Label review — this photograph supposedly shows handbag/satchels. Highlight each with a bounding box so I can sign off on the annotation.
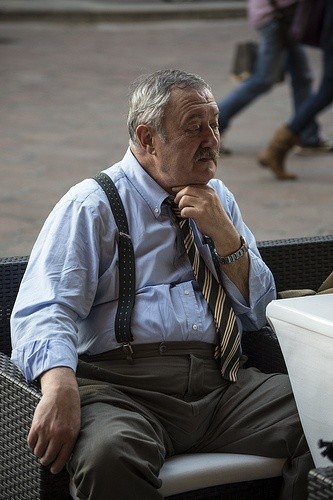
[230,42,286,83]
[290,0,325,48]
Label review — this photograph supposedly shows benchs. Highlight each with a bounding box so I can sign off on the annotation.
[0,231,332,500]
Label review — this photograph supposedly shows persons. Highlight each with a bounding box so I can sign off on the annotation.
[215,0,333,182]
[9,69,316,500]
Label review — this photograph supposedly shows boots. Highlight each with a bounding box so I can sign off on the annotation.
[256,123,297,180]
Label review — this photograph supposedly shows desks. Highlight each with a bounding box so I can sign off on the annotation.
[265,293,333,471]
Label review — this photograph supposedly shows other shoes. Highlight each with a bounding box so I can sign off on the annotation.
[295,140,333,155]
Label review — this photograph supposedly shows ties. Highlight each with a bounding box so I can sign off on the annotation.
[166,196,242,383]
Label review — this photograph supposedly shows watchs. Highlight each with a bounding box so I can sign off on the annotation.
[213,234,249,264]
